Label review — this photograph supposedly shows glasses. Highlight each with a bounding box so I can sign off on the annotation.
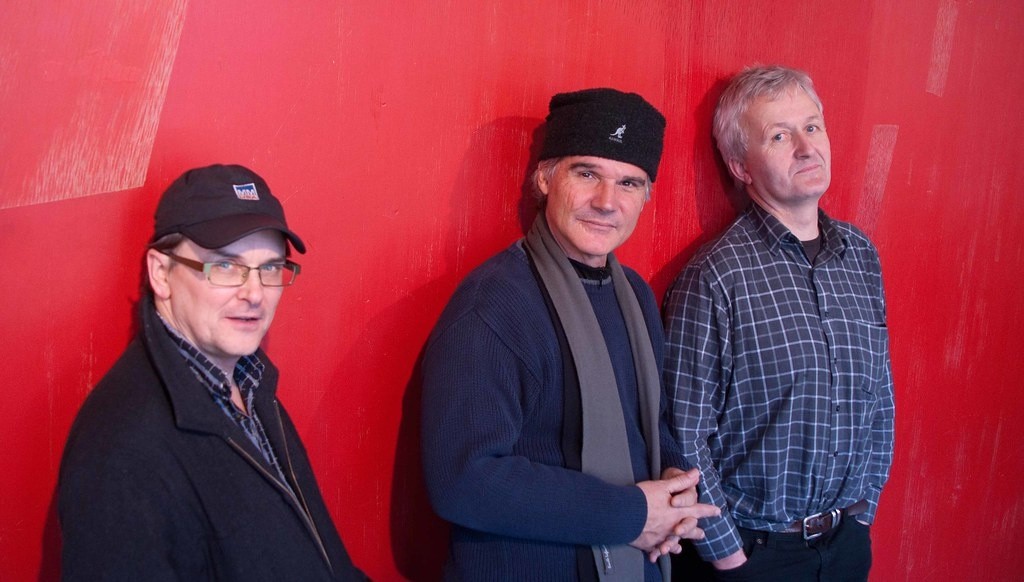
[160,251,301,287]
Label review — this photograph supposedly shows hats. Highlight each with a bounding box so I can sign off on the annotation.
[538,85,666,185]
[155,164,308,255]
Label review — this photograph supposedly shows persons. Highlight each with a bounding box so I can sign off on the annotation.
[56,162,373,582]
[664,66,896,582]
[391,86,722,582]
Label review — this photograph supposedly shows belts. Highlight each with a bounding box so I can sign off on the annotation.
[779,500,870,541]
